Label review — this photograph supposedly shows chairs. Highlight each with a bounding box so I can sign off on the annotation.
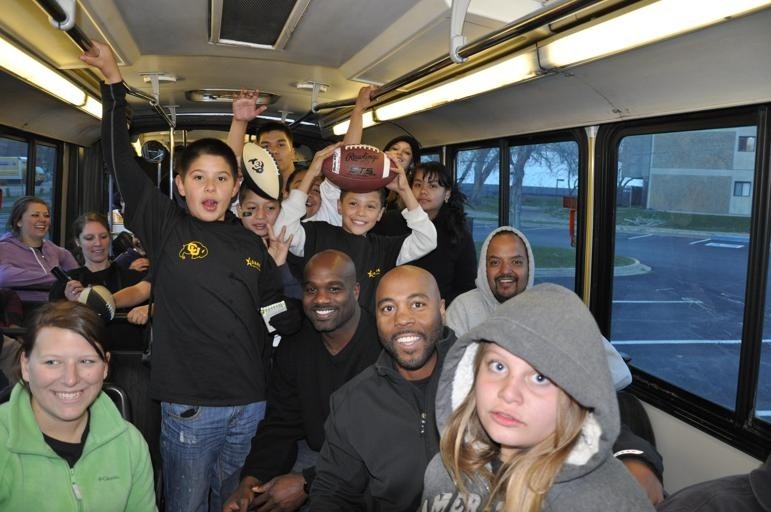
[1,348,162,512]
[110,313,151,351]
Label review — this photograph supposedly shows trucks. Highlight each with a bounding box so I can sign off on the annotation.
[1,157,45,186]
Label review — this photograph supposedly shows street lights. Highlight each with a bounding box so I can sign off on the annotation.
[554,177,565,201]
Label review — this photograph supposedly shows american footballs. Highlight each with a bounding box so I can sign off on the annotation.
[321,145,398,193]
[240,139,282,204]
[79,283,116,326]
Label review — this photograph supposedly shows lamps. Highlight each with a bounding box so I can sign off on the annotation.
[0,36,87,110]
[536,1,771,77]
[80,93,103,121]
[373,45,554,129]
[333,108,383,141]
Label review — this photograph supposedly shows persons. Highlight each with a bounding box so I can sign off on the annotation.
[656,454,771,512]
[598,328,664,507]
[420,282,658,512]
[444,224,535,341]
